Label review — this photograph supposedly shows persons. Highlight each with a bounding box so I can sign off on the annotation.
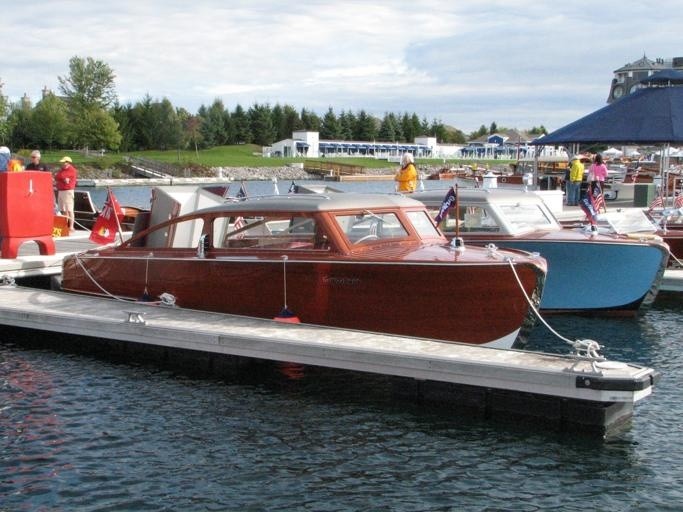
[568,155,587,206]
[589,154,608,194]
[25,149,49,171]
[0,143,11,171]
[55,156,77,232]
[395,152,417,194]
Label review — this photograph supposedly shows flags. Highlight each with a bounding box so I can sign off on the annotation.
[235,216,245,239]
[674,196,683,208]
[649,191,662,210]
[89,191,125,246]
[580,180,604,224]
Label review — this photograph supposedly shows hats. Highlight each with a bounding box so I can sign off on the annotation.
[571,156,578,160]
[59,156,72,163]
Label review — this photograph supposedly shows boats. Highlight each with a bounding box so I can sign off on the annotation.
[58,184,548,354]
[354,188,672,316]
